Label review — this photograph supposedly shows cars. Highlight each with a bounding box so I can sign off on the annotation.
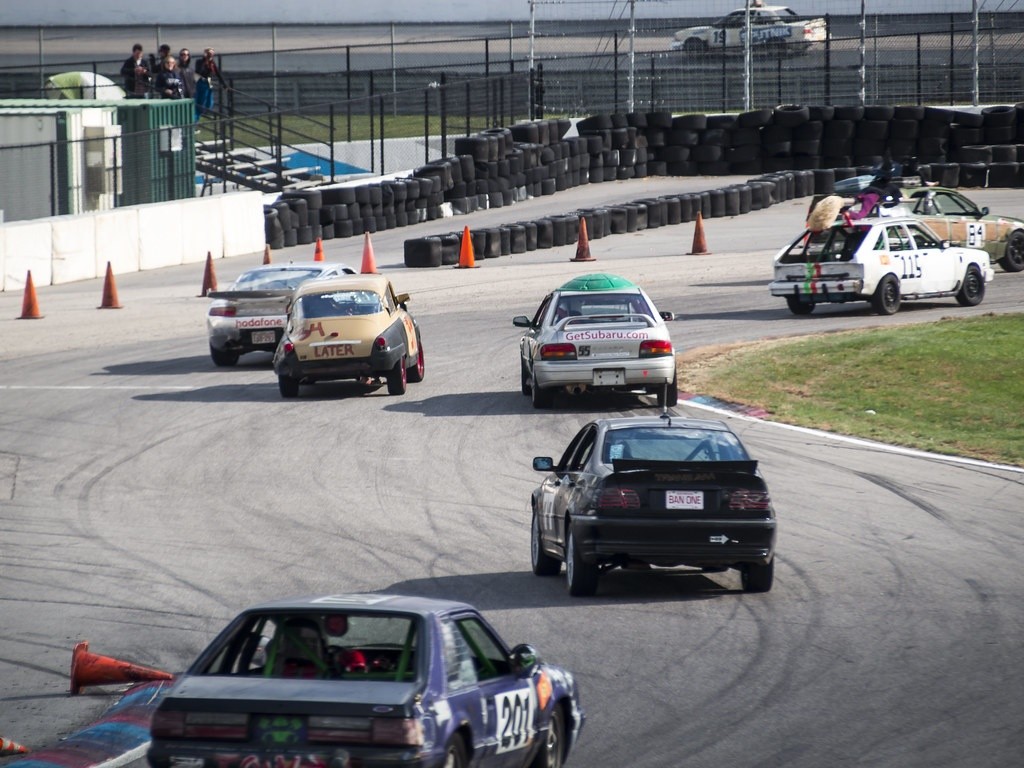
[512,288,678,407]
[201,260,358,367]
[672,0,833,58]
[272,272,424,398]
[145,593,587,766]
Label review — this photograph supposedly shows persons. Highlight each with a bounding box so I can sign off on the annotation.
[121,44,218,122]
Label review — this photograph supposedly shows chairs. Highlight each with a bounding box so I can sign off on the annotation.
[312,299,339,316]
[557,311,583,324]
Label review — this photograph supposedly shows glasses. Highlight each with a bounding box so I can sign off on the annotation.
[180,53,189,56]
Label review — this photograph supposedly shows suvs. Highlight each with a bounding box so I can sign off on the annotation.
[534,415,777,597]
[807,187,1023,272]
[768,218,995,316]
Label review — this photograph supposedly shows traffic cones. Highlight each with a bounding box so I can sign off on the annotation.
[69,641,172,697]
[96,261,124,309]
[314,236,323,261]
[454,226,481,268]
[262,243,272,265]
[15,269,45,320]
[196,251,218,297]
[360,231,377,274]
[685,210,712,256]
[568,217,597,261]
[0,737,31,756]
